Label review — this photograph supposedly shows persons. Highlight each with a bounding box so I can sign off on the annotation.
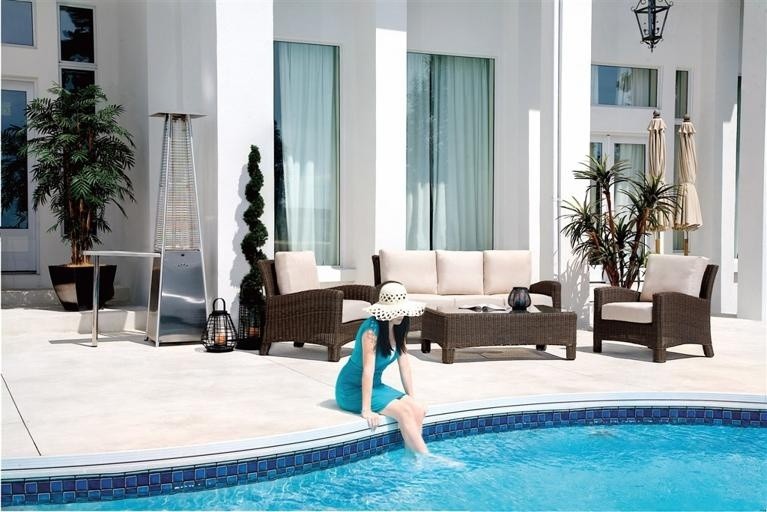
[336,282,466,468]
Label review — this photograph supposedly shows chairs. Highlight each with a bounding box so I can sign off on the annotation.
[591,247,719,367]
[256,249,383,360]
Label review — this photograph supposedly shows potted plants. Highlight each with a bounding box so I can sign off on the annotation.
[4,76,133,310]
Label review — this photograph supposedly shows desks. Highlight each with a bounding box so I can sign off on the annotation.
[81,245,162,347]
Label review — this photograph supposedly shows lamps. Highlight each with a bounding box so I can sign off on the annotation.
[630,1,675,54]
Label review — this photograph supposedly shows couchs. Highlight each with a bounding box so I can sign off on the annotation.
[373,248,562,344]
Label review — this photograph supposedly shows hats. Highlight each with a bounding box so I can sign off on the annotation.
[363,283,427,322]
